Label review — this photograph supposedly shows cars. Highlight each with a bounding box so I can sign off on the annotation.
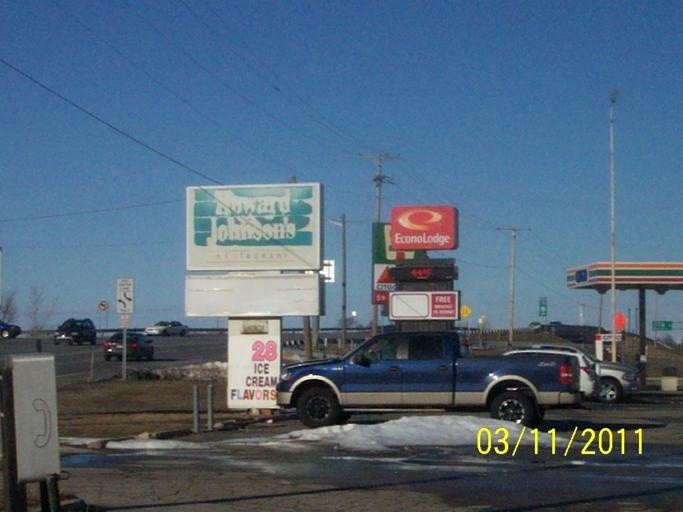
[104,333,154,361]
[145,319,190,337]
[504,342,643,403]
[0,319,21,338]
[55,318,97,346]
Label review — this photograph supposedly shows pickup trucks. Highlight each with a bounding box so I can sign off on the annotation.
[277,330,579,427]
